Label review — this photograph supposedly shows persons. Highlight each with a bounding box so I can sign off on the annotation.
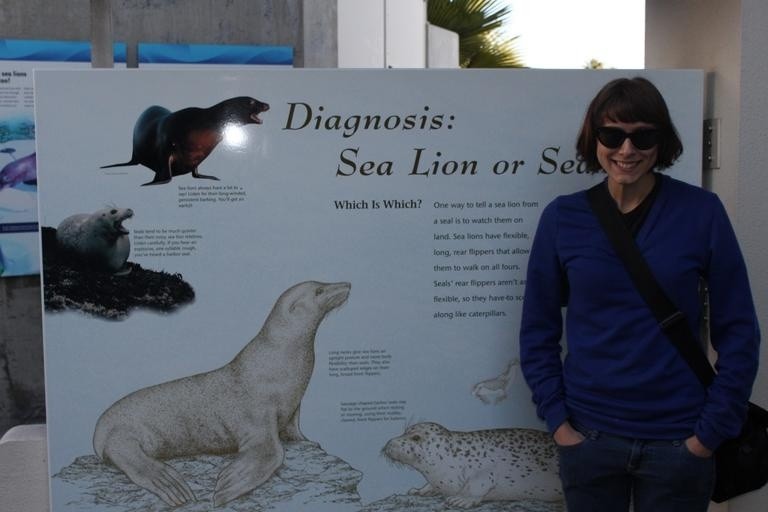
[516,76,765,511]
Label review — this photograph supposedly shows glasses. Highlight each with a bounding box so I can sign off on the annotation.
[595,125,657,150]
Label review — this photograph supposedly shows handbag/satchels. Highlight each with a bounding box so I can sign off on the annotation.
[710,398,768,504]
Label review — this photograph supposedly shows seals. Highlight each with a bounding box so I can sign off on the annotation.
[383,421,566,510]
[54,207,134,286]
